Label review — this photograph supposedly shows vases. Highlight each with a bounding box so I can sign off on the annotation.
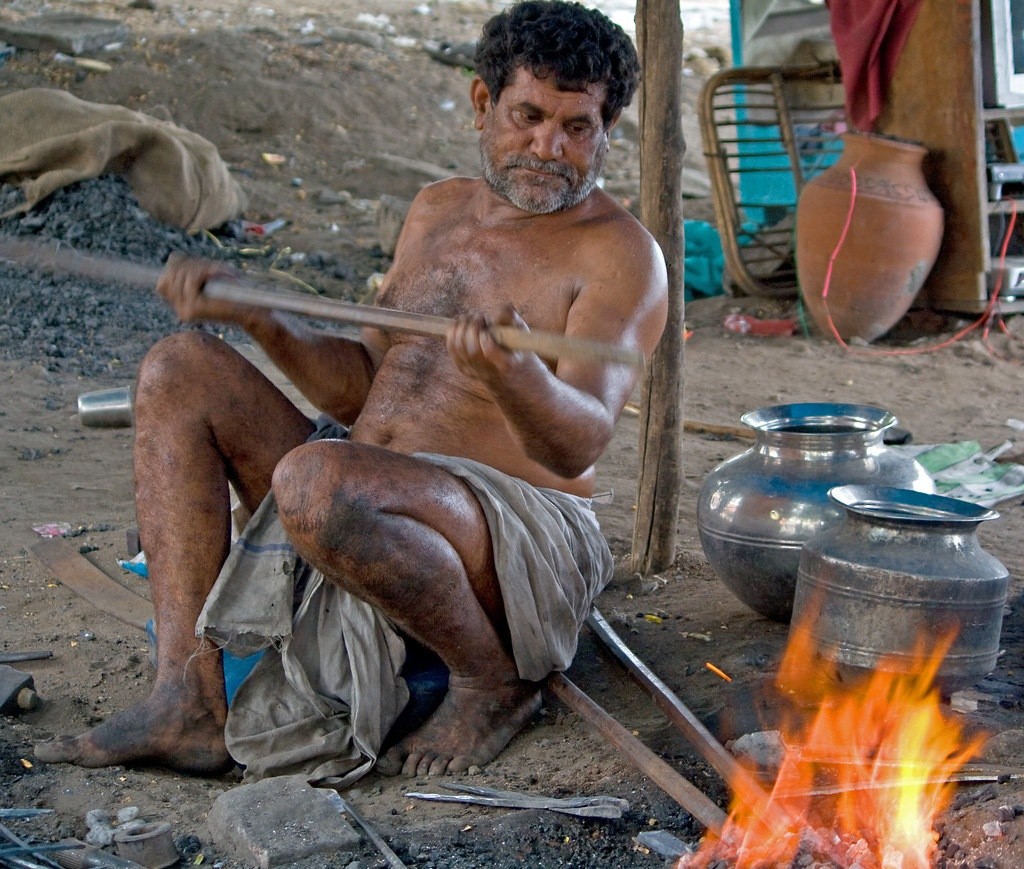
[697,403,938,625]
[791,484,1010,698]
[794,132,945,343]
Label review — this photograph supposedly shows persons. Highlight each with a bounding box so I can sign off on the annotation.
[32,0,669,778]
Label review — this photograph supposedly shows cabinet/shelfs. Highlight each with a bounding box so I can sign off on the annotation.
[825,0,1024,313]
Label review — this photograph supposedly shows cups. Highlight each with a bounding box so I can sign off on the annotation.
[78,386,133,427]
